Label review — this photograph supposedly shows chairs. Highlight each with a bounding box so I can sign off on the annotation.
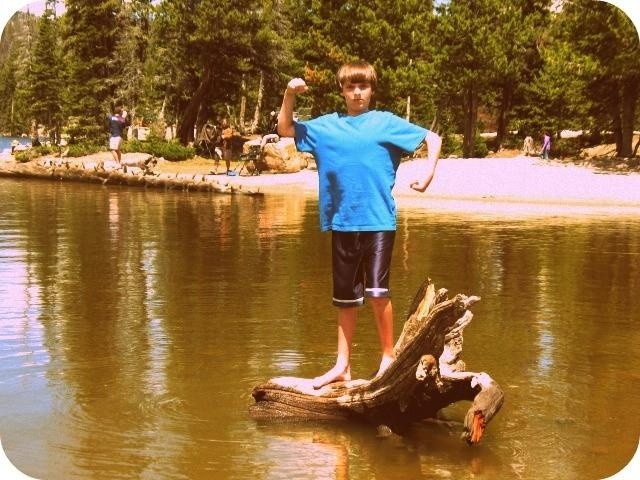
[198,123,221,159]
[237,142,262,177]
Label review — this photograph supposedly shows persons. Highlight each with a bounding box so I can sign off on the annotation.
[259,111,279,152]
[210,117,233,175]
[541,131,551,161]
[109,107,127,170]
[277,60,443,390]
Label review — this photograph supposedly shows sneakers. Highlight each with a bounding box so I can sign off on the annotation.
[210,169,218,176]
[112,162,125,170]
